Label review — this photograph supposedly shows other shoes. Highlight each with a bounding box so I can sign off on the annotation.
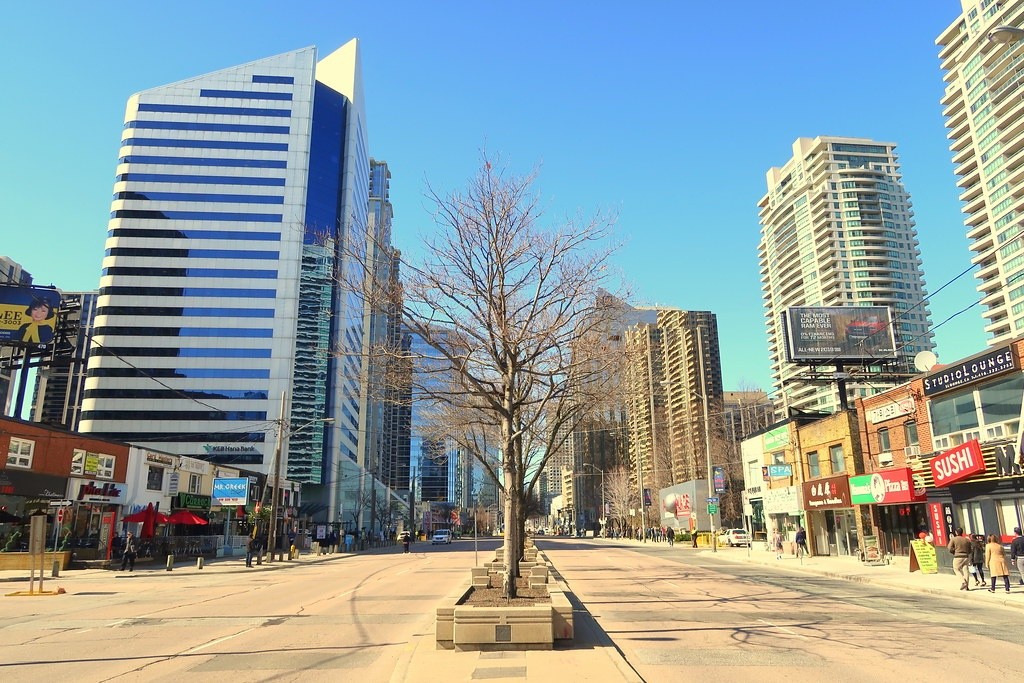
[1006,590,1010,594]
[960,582,967,590]
[975,580,980,586]
[964,586,969,591]
[981,581,986,586]
[988,589,995,593]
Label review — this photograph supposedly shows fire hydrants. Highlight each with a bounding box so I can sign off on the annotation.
[290,544,296,559]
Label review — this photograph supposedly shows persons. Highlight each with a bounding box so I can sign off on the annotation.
[647,527,674,547]
[691,527,697,548]
[348,527,372,544]
[111,532,120,559]
[969,534,986,586]
[874,477,884,501]
[985,534,1010,594]
[403,533,412,554]
[948,527,971,591]
[775,529,782,560]
[796,527,812,558]
[246,533,254,567]
[1011,527,1024,585]
[289,527,296,551]
[607,527,642,542]
[383,528,396,541]
[120,531,137,571]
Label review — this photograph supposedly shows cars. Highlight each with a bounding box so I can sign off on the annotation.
[431,528,453,545]
[722,528,753,548]
[397,530,411,544]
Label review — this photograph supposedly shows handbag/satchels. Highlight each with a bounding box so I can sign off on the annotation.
[968,564,976,573]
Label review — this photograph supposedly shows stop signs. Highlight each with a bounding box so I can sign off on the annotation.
[57,509,64,522]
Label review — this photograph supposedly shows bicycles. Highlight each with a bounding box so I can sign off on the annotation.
[797,542,805,565]
[775,543,782,560]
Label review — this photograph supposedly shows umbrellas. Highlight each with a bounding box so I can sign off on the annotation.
[120,510,168,523]
[140,502,154,539]
[169,510,208,525]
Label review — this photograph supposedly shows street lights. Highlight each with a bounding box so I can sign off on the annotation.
[265,417,336,559]
[410,467,439,544]
[582,462,606,540]
[609,431,647,544]
[659,380,717,553]
